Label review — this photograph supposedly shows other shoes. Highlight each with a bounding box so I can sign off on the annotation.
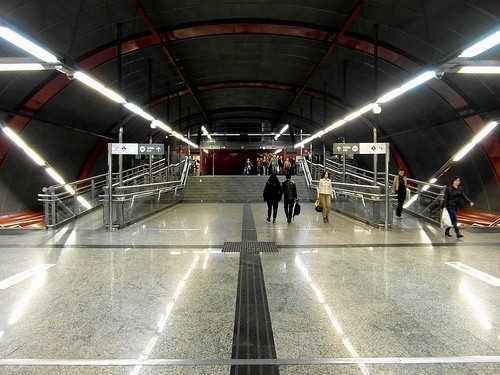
[266,217,271,222]
[456,231,463,238]
[395,215,403,219]
[273,219,276,223]
[287,217,292,223]
[257,174,261,176]
[445,230,452,236]
[323,216,329,223]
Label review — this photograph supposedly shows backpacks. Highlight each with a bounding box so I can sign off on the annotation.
[293,201,301,222]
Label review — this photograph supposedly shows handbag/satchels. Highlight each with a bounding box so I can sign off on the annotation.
[441,208,452,228]
[315,205,322,212]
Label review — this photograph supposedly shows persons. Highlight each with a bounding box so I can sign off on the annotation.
[244,154,297,175]
[393,168,407,218]
[263,173,298,224]
[441,176,474,239]
[319,171,333,224]
